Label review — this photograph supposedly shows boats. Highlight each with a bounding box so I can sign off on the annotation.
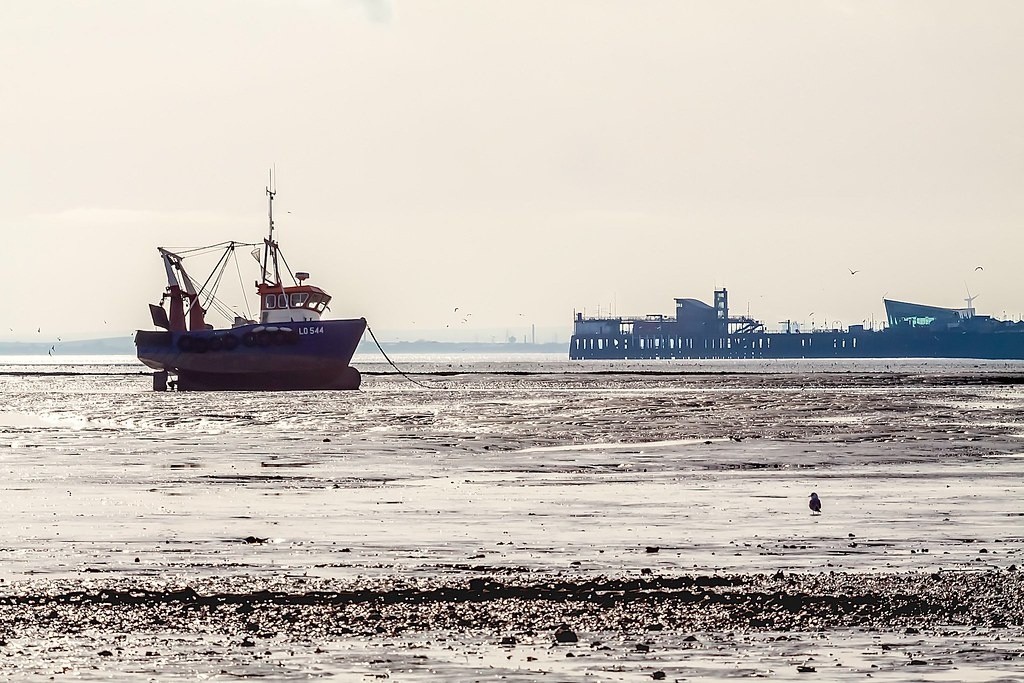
[131,164,372,391]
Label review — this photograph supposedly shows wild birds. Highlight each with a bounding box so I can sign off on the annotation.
[808,493,822,515]
